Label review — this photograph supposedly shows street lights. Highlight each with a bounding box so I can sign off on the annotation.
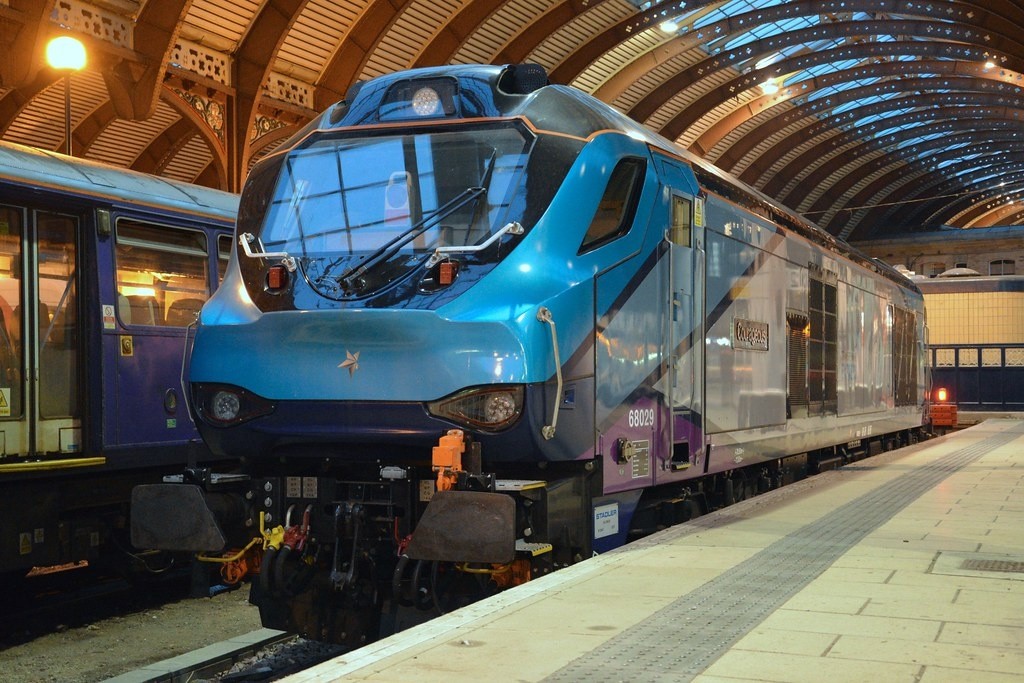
[47,37,88,155]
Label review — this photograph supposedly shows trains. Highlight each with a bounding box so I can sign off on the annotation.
[184,64,932,643]
[0,138,242,609]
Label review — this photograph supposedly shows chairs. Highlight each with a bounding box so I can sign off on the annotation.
[14,290,201,340]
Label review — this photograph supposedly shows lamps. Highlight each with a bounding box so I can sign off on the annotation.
[764,77,778,92]
[662,19,680,32]
